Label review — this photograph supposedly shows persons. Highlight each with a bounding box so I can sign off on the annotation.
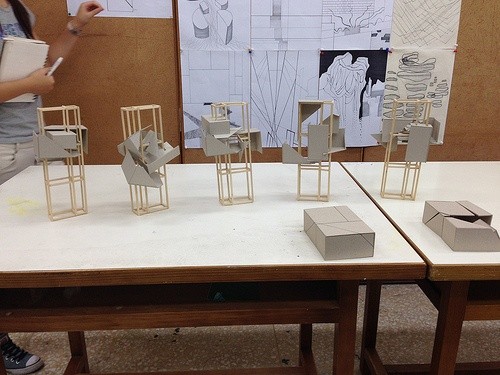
[0,0,105,375]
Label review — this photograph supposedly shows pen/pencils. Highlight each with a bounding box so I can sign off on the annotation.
[47,57,63,76]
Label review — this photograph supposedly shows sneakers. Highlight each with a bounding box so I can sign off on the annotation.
[0,333,43,374]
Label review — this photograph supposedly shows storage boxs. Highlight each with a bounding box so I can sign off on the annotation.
[422,199,500,252]
[303,205,376,260]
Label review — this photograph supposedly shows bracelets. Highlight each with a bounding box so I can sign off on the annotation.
[68,21,81,35]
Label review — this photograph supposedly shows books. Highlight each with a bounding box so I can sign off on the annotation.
[0,34,50,102]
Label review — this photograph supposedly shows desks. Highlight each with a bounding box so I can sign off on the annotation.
[0,160,500,375]
